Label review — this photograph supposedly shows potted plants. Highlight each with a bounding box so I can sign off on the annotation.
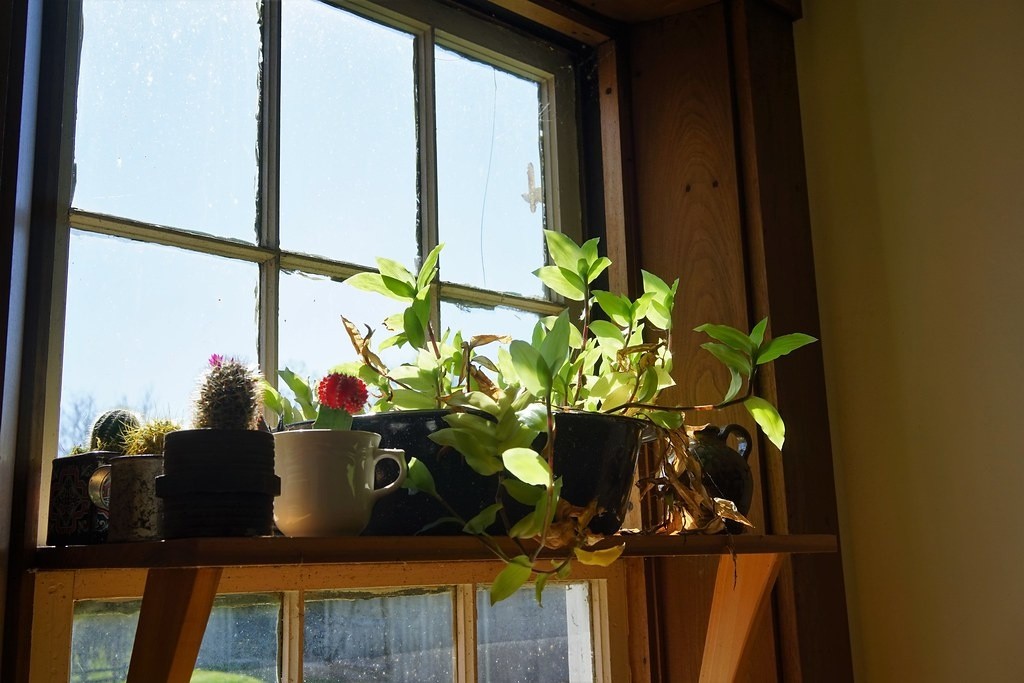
[46,230,820,609]
